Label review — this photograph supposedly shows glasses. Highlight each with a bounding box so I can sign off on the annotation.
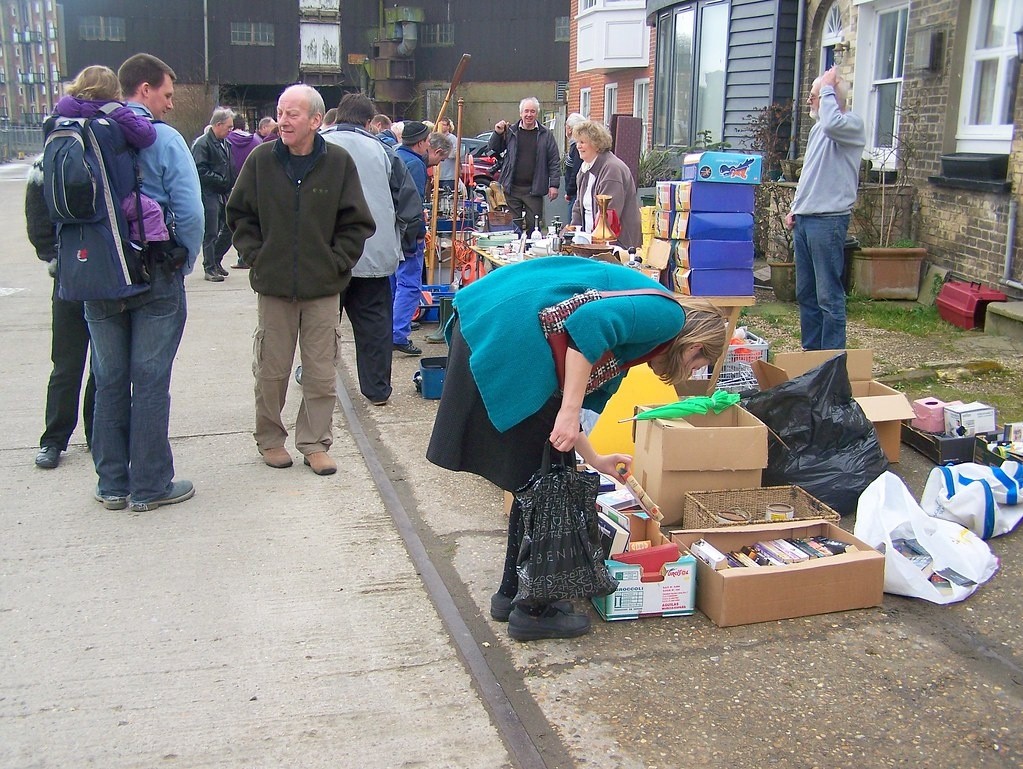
[807,95,820,102]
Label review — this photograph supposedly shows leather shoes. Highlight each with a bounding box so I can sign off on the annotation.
[392,340,422,354]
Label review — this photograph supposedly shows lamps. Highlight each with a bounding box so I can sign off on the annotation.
[831,42,852,65]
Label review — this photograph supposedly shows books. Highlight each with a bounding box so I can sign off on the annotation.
[691,536,852,569]
[584,469,678,582]
[619,468,664,521]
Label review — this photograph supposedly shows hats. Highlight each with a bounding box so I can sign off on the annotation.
[402,122,430,144]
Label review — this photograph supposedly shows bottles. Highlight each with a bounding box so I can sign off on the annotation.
[562,233,574,256]
[530,215,562,252]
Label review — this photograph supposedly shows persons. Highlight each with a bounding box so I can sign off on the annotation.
[489,98,560,231]
[786,66,866,351]
[565,113,588,224]
[392,118,458,354]
[192,109,236,282]
[318,93,423,404]
[227,114,260,268]
[85,53,205,512]
[427,256,727,638]
[225,85,377,474]
[24,65,121,468]
[572,122,641,249]
[254,117,280,143]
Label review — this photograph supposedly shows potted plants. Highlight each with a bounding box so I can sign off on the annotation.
[738,97,804,301]
[847,85,930,302]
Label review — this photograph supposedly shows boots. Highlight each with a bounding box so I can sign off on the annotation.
[424,297,453,343]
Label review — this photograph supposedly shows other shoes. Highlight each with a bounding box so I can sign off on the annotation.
[295,366,302,386]
[216,263,229,276]
[230,262,243,268]
[205,272,224,282]
[508,606,592,640]
[491,592,573,622]
[130,239,151,284]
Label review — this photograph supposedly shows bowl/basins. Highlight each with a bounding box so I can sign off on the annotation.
[571,244,614,257]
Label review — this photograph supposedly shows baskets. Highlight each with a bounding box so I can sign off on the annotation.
[716,332,769,378]
[681,485,841,528]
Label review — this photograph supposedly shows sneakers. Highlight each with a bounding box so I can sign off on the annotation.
[258,444,293,468]
[130,480,195,512]
[94,484,127,509]
[36,446,61,468]
[304,452,337,475]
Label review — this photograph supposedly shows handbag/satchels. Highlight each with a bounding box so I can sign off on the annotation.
[511,439,619,605]
[854,472,996,604]
[538,289,687,398]
[919,460,1023,541]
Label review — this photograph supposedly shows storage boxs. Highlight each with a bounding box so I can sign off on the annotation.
[585,349,1021,630]
[437,219,471,231]
[934,278,1006,329]
[634,150,762,297]
[418,283,457,323]
